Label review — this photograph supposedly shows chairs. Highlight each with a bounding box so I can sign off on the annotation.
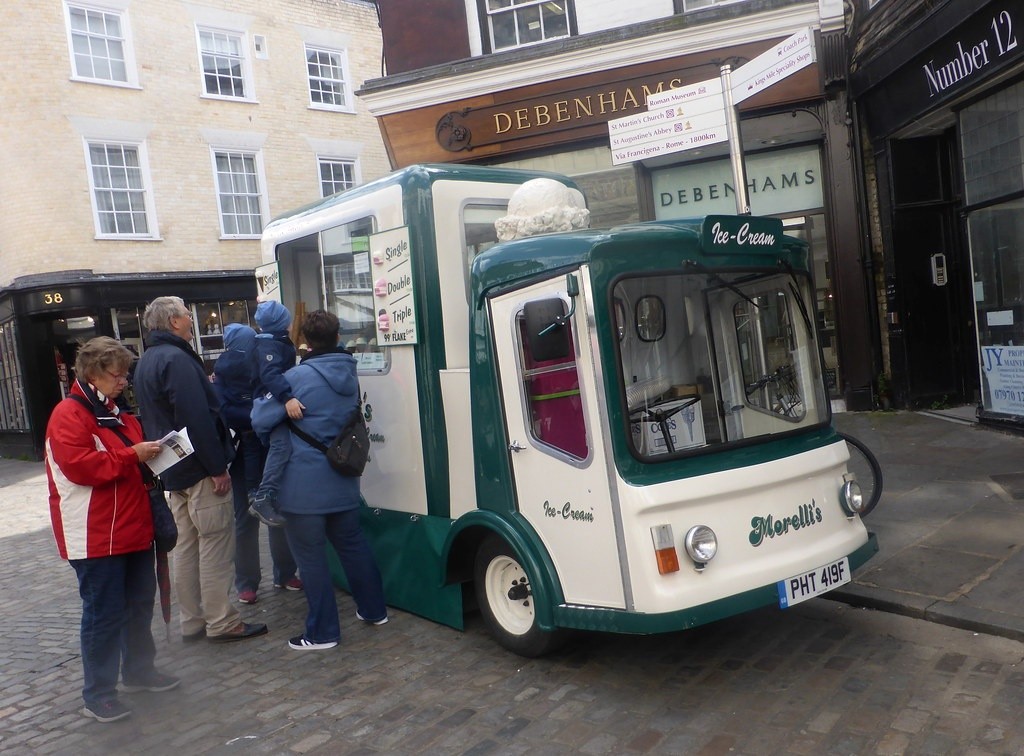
[529,317,587,459]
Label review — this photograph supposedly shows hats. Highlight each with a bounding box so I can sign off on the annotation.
[254,300,292,332]
[223,323,258,351]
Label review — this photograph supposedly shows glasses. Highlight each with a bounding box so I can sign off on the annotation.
[102,366,129,383]
[184,311,193,319]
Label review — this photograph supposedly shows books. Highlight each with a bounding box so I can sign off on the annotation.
[145,427,194,475]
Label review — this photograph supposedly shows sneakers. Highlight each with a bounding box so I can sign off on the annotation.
[248,489,288,527]
[247,489,280,510]
[121,666,181,692]
[356,608,388,625]
[83,692,132,722]
[274,576,303,591]
[238,585,258,603]
[288,633,340,650]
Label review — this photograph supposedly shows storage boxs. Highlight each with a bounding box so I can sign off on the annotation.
[630,383,720,457]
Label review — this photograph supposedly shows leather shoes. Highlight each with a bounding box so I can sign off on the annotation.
[182,621,206,641]
[207,622,268,643]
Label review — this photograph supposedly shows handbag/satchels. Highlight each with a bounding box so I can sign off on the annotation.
[148,487,178,552]
[325,409,371,477]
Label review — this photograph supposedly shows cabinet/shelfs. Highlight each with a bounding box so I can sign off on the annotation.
[199,334,228,361]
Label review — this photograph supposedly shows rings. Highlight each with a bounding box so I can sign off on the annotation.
[220,485,225,488]
[152,453,155,458]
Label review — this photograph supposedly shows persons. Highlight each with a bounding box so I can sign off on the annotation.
[44,335,183,722]
[209,323,302,603]
[215,301,305,528]
[133,296,269,641]
[250,309,388,650]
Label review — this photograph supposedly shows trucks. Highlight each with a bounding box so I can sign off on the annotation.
[256,161,882,661]
